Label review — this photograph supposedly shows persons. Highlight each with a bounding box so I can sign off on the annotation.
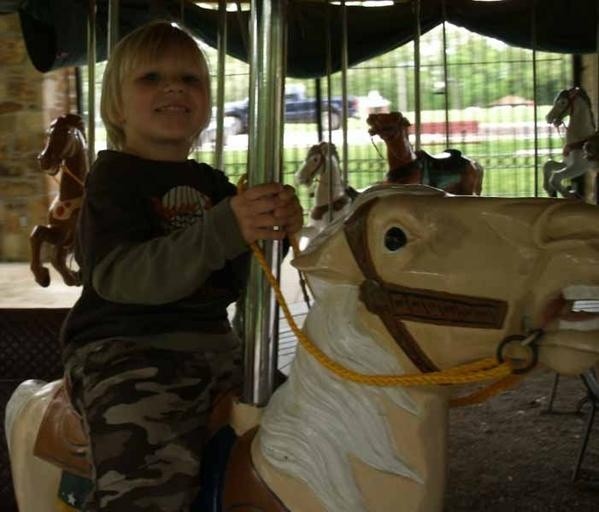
[55,17,307,511]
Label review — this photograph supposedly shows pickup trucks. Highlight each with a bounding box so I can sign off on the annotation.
[223,86,358,135]
